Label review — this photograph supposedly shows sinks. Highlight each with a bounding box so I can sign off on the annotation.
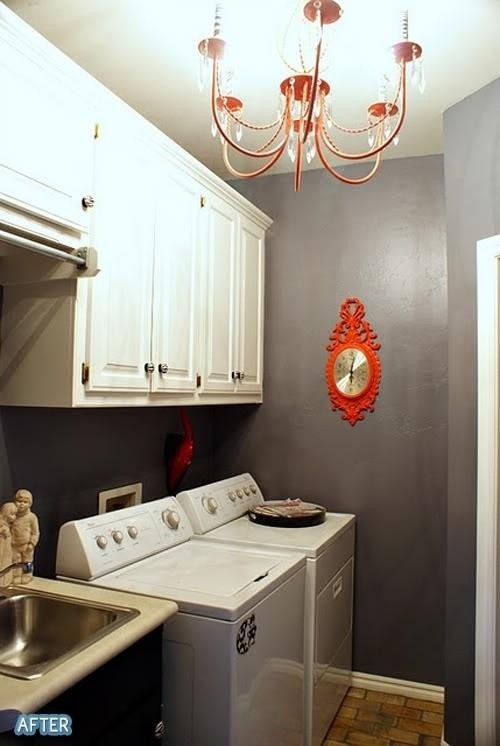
[0,584,142,682]
[0,561,34,578]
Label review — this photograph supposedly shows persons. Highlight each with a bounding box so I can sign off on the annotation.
[9,486,40,585]
[0,501,18,588]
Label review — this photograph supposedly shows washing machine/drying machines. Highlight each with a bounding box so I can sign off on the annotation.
[175,473,358,746]
[55,496,308,746]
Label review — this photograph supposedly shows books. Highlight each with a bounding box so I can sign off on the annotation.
[250,496,324,518]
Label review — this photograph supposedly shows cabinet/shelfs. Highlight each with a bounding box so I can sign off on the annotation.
[0,83,273,410]
[0,0,91,249]
[27,626,164,746]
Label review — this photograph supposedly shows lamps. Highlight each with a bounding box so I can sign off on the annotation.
[196,0,422,192]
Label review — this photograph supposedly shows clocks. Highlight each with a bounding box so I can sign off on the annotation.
[324,297,380,426]
